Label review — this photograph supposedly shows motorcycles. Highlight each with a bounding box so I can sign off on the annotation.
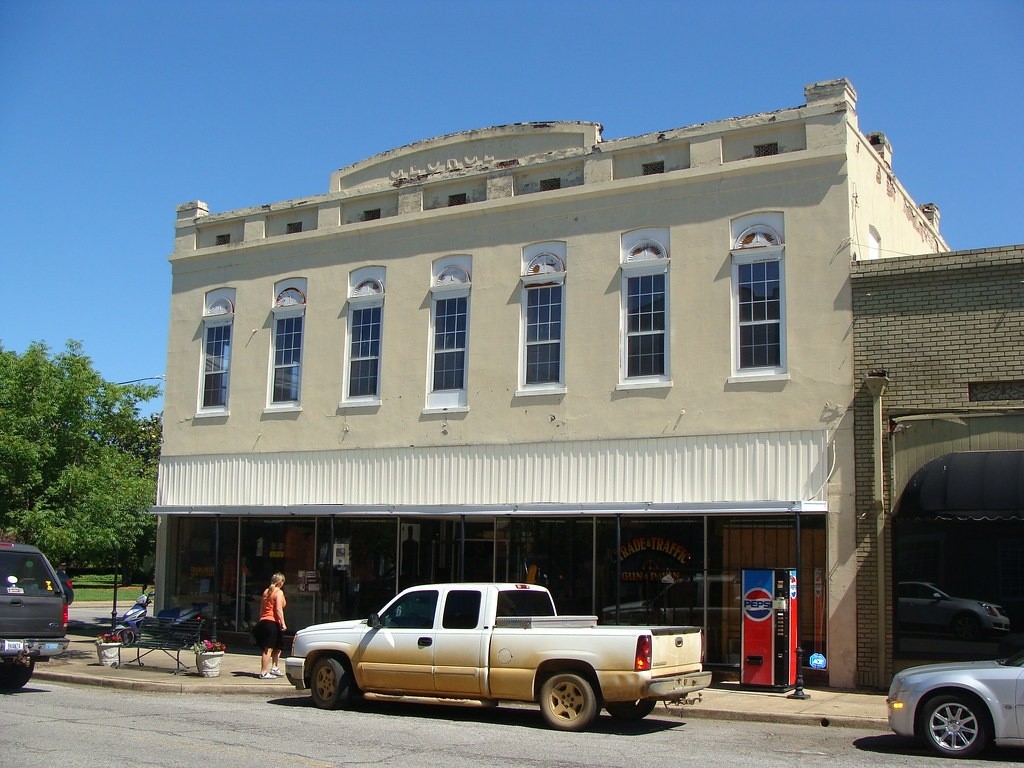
[119,583,210,646]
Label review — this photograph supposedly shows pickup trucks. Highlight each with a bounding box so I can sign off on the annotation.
[284,581,713,730]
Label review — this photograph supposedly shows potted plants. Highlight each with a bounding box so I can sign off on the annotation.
[192,639,227,678]
[95,633,124,668]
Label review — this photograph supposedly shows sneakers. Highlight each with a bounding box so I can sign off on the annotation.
[259,672,277,679]
[270,668,285,676]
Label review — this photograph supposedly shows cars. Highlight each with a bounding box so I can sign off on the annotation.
[885,651,1024,756]
[893,580,1012,647]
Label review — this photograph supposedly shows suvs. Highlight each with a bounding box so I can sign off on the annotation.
[0,542,71,692]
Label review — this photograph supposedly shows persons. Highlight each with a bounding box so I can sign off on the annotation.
[257,572,287,679]
